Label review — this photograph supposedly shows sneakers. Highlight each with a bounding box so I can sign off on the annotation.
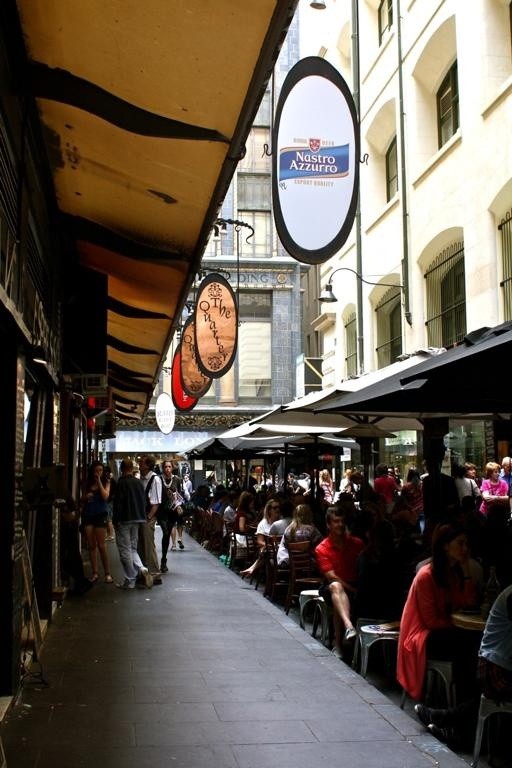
[138,567,153,589]
[178,539,184,549]
[171,545,176,550]
[414,704,462,753]
[332,647,344,658]
[116,583,134,588]
[344,627,357,640]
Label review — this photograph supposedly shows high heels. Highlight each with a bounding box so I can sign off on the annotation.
[240,570,251,579]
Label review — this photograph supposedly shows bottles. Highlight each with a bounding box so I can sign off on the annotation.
[417,512,426,536]
[480,566,501,620]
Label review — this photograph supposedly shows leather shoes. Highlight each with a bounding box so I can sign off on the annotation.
[90,572,99,583]
[104,573,114,583]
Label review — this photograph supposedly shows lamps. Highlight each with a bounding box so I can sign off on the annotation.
[319,268,405,301]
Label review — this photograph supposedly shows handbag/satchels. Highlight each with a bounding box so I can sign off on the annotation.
[276,535,290,570]
[182,502,195,516]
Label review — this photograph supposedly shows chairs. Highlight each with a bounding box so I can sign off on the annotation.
[186,500,511,768]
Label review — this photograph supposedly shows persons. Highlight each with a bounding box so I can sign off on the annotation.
[269,455,512,752]
[70,456,305,589]
